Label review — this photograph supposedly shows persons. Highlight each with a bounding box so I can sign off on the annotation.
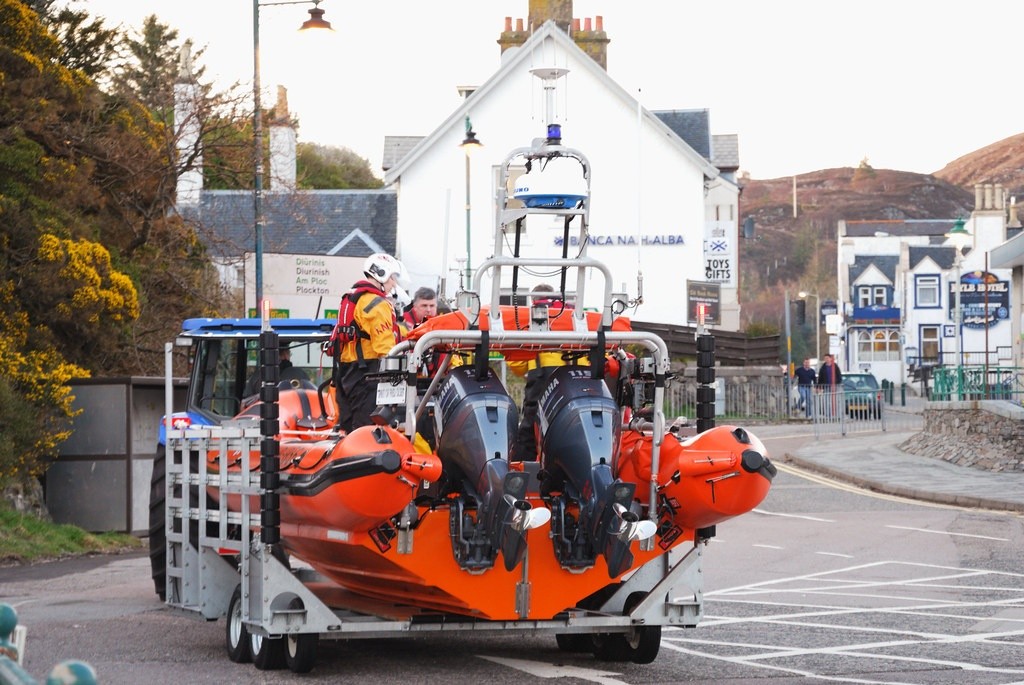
[323,252,410,436]
[278,345,294,371]
[782,354,842,418]
[397,284,453,379]
[507,283,613,376]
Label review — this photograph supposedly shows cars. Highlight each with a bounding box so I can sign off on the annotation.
[841,371,882,420]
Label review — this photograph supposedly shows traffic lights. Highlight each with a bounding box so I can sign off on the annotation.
[697,304,706,333]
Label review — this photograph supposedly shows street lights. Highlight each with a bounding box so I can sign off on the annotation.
[253,0,333,317]
[798,292,821,364]
[461,116,483,293]
[944,217,973,367]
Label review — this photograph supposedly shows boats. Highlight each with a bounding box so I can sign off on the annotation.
[205,143,778,621]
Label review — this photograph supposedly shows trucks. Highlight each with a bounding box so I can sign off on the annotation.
[148,317,716,674]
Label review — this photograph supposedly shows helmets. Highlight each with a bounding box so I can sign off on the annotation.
[363,253,400,284]
